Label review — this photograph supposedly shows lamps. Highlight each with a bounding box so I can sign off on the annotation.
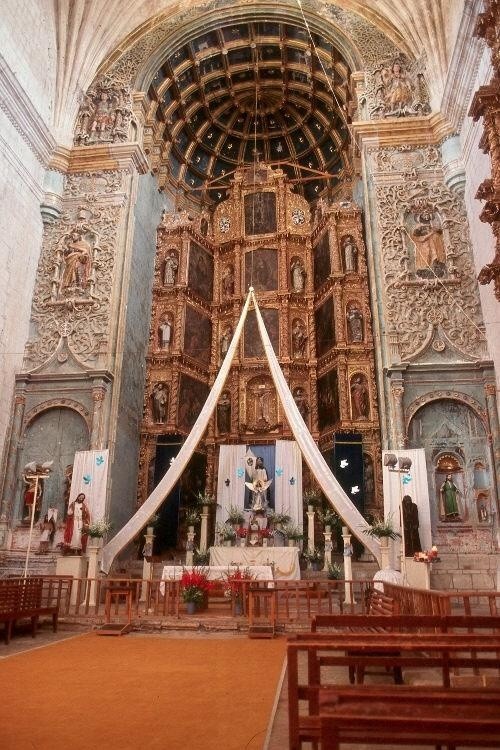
[383,453,413,474]
[24,459,56,576]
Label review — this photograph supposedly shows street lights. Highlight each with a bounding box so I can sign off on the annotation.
[381,453,411,587]
[19,458,54,608]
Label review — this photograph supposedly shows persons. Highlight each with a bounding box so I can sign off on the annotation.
[438,473,461,520]
[413,205,447,271]
[87,88,117,135]
[343,236,365,420]
[218,266,233,430]
[384,62,413,111]
[292,259,307,419]
[60,228,91,291]
[20,472,42,523]
[63,492,91,552]
[246,457,273,504]
[34,513,55,554]
[250,256,268,421]
[154,252,178,422]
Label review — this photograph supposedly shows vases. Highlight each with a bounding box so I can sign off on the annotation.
[236,606,242,615]
[187,602,195,614]
[92,539,99,546]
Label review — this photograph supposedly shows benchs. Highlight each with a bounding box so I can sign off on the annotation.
[285,588,500,750]
[0,576,61,645]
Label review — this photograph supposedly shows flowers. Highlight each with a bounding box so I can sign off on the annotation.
[412,545,439,565]
[143,486,402,605]
[77,512,114,539]
